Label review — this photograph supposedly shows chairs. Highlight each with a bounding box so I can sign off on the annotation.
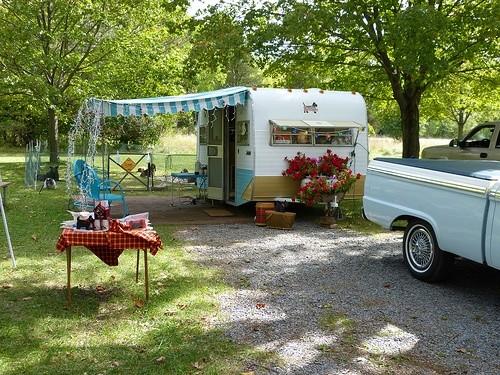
[72,158,127,219]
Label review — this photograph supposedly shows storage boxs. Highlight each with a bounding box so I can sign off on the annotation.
[255,202,275,226]
[264,210,296,230]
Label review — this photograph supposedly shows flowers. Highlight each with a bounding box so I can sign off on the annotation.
[282,150,361,206]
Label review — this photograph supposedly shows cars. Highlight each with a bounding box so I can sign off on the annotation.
[421,121,500,160]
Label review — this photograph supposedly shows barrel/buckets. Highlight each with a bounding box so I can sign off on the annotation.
[256,203,275,225]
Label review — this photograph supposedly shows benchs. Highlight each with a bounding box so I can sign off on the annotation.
[0,181,11,203]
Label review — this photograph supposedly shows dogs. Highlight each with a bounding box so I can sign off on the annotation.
[180,168,195,183]
[138,163,156,178]
[77,215,95,230]
[34,165,65,181]
[274,200,289,213]
[43,177,59,190]
[303,102,317,113]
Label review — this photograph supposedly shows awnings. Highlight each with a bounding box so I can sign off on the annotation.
[85,87,250,213]
[270,119,366,145]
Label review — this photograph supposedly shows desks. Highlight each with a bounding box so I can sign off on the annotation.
[170,170,207,207]
[56,217,163,307]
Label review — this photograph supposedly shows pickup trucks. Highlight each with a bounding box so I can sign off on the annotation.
[362,157,500,282]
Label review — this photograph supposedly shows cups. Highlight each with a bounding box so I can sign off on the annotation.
[199,170,207,174]
[102,220,109,230]
[94,220,100,231]
